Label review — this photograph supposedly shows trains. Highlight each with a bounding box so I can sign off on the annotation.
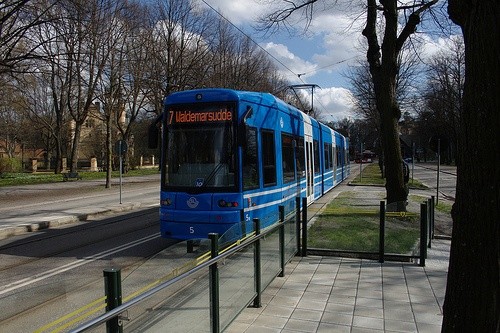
[157,88,350,253]
